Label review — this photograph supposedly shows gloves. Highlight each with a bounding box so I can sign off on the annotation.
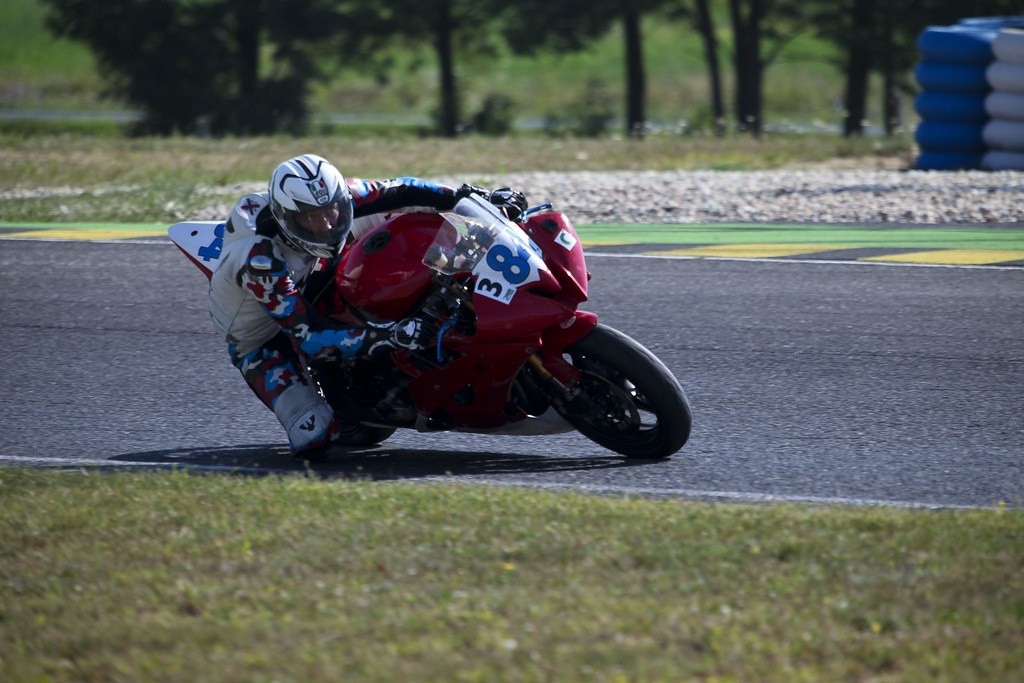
[455,182,528,219]
[363,317,431,356]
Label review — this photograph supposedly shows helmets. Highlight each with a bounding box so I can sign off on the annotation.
[268,154,353,258]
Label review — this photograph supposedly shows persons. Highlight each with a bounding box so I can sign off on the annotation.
[207,153,527,460]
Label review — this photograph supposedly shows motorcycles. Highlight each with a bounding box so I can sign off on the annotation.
[168,193,695,460]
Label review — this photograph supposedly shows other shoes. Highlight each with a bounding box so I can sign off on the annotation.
[334,417,395,446]
